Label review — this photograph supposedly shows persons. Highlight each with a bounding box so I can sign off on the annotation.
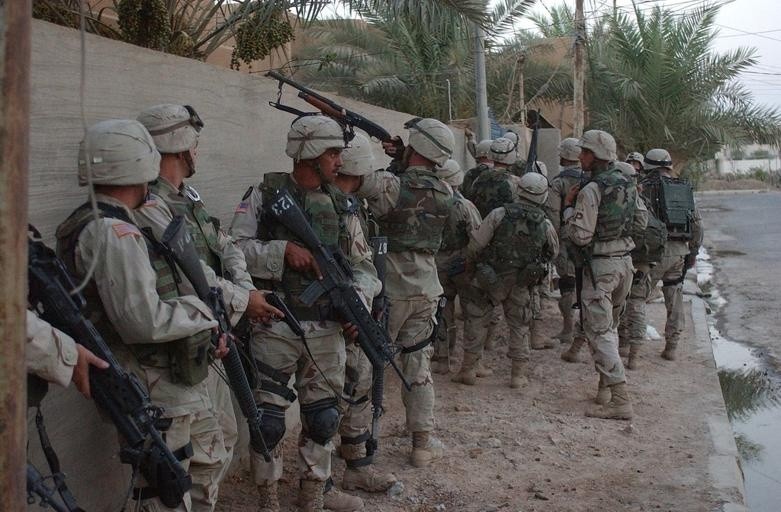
[58,118,233,511]
[322,133,396,511]
[228,114,379,510]
[336,118,456,469]
[26,309,111,400]
[138,102,287,466]
[439,128,704,420]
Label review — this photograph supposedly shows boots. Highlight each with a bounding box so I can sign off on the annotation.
[255,479,280,512]
[619,329,643,370]
[431,323,494,385]
[531,317,585,362]
[412,431,446,467]
[343,464,397,491]
[661,342,677,360]
[511,359,530,390]
[585,372,634,420]
[297,478,327,512]
[323,477,364,512]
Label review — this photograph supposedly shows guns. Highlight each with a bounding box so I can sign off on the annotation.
[262,190,414,394]
[27,224,192,507]
[162,215,273,464]
[524,109,541,176]
[268,69,406,160]
[363,236,392,462]
[573,181,587,332]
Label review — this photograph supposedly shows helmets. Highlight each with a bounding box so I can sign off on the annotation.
[516,161,549,205]
[340,132,373,177]
[78,119,161,186]
[409,118,455,167]
[435,159,465,187]
[559,130,672,183]
[285,116,345,159]
[476,133,519,165]
[136,105,200,154]
